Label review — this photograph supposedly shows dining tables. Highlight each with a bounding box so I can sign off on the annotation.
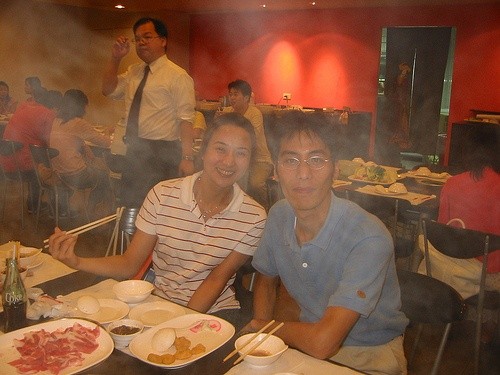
[0,267,378,375]
[0,112,114,149]
[264,162,454,263]
[199,101,315,113]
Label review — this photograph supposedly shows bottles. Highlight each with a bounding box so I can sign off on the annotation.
[2,258,28,333]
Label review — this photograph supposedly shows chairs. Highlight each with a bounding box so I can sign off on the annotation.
[27,142,95,233]
[396,269,466,375]
[249,159,275,208]
[421,218,500,375]
[0,138,35,232]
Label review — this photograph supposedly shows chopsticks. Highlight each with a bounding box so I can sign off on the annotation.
[223,319,284,365]
[42,214,117,248]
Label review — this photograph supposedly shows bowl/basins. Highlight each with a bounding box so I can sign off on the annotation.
[114,279,155,303]
[18,247,41,265]
[234,332,288,366]
[389,182,408,194]
[415,167,431,177]
[106,319,144,346]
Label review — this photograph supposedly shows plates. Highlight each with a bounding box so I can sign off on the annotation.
[26,256,44,269]
[348,174,396,185]
[129,302,185,329]
[73,298,129,325]
[375,188,399,195]
[0,317,114,375]
[128,313,235,367]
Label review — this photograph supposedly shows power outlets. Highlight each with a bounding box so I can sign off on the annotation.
[282,93,292,100]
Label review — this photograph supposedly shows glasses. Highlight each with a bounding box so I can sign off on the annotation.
[277,157,331,169]
[131,34,160,43]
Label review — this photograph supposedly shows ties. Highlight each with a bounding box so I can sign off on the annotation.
[125,66,150,147]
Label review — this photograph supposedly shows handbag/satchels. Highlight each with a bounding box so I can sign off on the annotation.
[417,217,483,300]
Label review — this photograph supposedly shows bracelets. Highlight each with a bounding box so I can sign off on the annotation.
[182,155,196,161]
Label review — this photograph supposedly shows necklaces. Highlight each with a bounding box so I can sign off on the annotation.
[195,179,226,219]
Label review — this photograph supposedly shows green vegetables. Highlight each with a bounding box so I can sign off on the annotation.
[353,164,385,183]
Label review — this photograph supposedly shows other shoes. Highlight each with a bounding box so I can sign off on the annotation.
[50,209,79,219]
[28,201,47,213]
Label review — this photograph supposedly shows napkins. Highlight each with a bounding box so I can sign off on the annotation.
[402,170,451,183]
[223,348,366,375]
[0,241,80,294]
[381,165,402,171]
[332,179,353,189]
[354,185,436,207]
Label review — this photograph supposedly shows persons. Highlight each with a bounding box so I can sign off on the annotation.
[211,79,274,212]
[49,113,266,331]
[235,111,410,375]
[439,119,500,352]
[100,18,194,235]
[0,75,207,227]
